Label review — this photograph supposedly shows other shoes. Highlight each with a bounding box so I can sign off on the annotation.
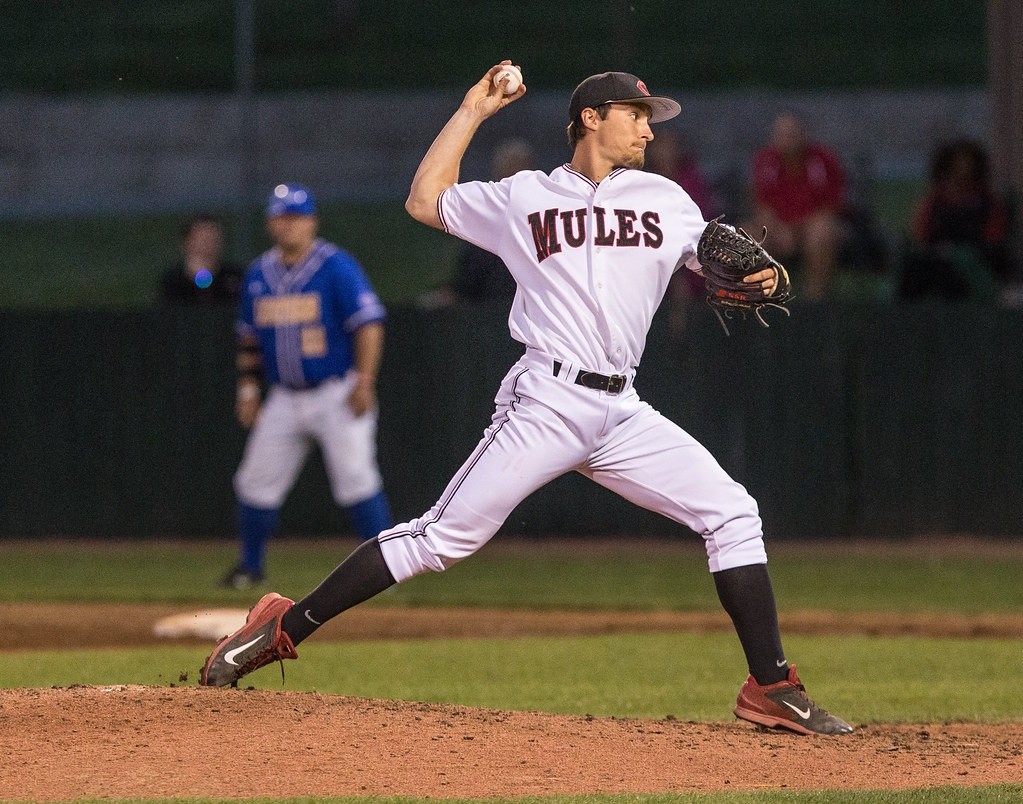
[218,562,265,587]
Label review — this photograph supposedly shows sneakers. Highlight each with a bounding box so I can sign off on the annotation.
[198,592,298,687]
[733,664,854,739]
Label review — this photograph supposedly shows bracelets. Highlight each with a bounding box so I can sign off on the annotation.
[234,382,260,401]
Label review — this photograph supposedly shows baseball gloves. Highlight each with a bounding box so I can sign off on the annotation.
[697,216,790,337]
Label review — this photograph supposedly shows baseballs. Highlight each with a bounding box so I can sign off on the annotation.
[493,64,523,96]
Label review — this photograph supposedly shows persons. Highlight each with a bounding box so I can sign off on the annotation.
[647,132,727,298]
[894,133,1006,304]
[417,137,545,308]
[216,182,393,593]
[748,107,844,304]
[158,213,244,306]
[198,59,856,740]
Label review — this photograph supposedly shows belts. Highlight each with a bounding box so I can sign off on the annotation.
[553,360,633,396]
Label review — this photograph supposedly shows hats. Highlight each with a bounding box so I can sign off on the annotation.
[567,72,681,124]
[264,184,314,214]
